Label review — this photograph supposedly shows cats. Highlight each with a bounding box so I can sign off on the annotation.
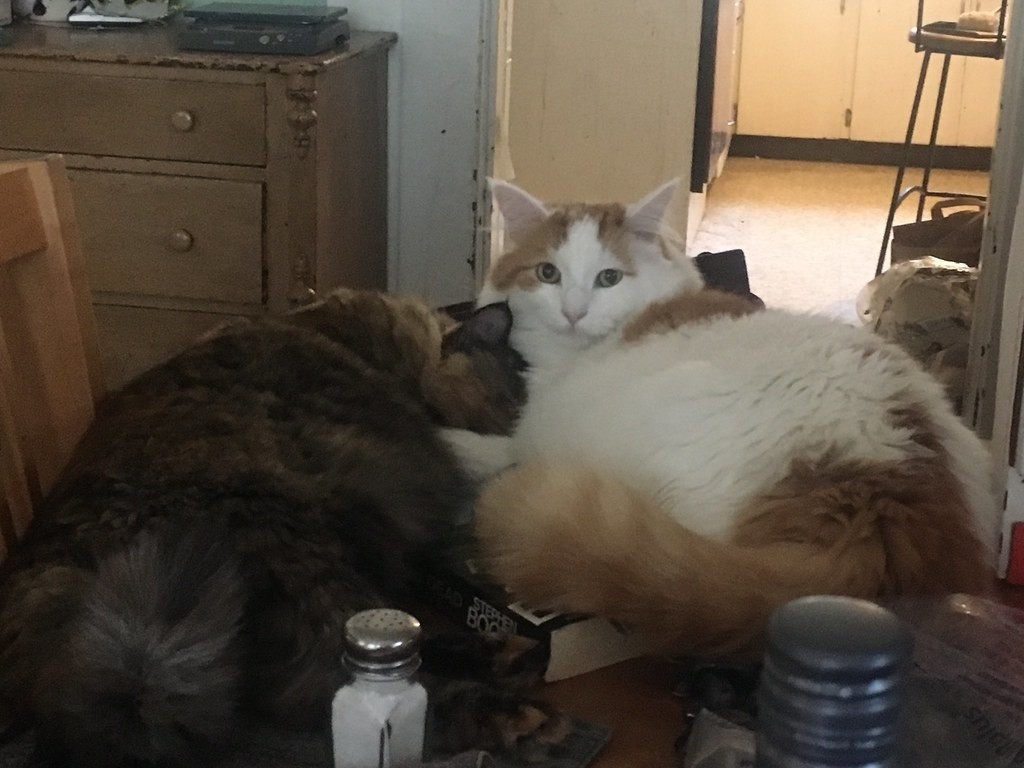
[441,174,1001,667]
[0,289,529,768]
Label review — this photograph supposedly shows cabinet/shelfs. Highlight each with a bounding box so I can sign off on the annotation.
[0,21,397,396]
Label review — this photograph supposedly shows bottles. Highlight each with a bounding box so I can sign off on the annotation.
[755,595,913,768]
[331,609,427,768]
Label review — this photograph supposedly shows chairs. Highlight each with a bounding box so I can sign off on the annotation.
[0,154,91,549]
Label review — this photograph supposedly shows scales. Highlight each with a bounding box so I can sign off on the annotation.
[176,4,350,56]
